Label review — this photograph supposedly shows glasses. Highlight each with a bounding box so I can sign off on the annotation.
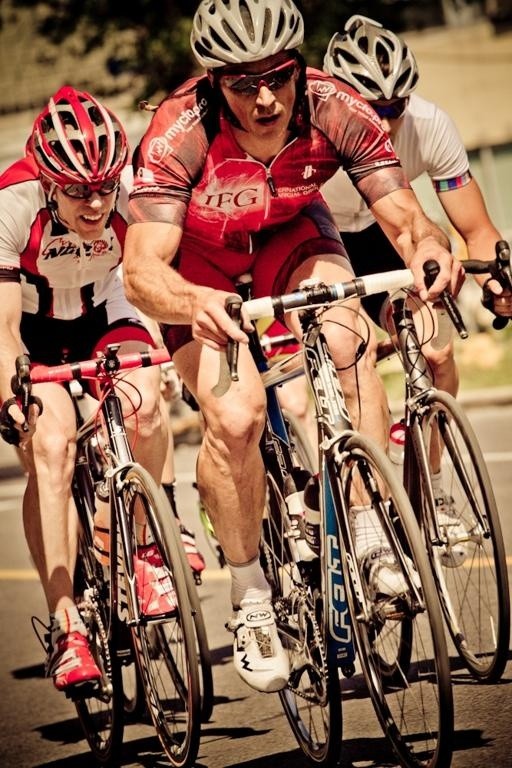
[41,170,120,199]
[223,59,301,95]
[370,95,410,120]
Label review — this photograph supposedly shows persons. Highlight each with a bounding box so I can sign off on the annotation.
[0,0,512,691]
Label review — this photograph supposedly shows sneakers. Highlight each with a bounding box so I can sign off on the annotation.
[225,597,290,693]
[292,512,319,561]
[180,524,205,572]
[434,489,468,567]
[49,619,102,691]
[132,542,178,615]
[349,501,422,597]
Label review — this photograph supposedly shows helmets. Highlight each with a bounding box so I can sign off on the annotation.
[323,15,419,101]
[26,86,128,184]
[190,0,305,69]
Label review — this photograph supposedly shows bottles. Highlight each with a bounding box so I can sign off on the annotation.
[388,419,409,466]
[302,475,321,551]
[92,481,111,567]
